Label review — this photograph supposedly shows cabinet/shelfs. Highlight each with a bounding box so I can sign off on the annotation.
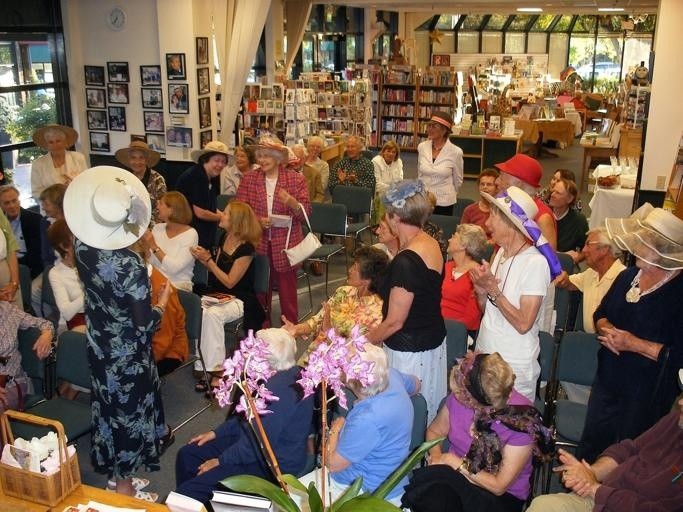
[282,79,372,157]
[415,66,457,151]
[449,129,524,181]
[378,64,418,152]
[235,81,319,148]
[622,73,651,129]
[352,64,379,148]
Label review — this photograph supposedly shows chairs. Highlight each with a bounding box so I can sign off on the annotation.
[534,331,556,495]
[224,255,271,349]
[9,331,91,443]
[454,198,475,218]
[324,184,373,261]
[272,269,313,323]
[424,215,459,261]
[18,327,52,410]
[304,203,349,299]
[158,288,213,433]
[592,119,602,130]
[546,331,602,494]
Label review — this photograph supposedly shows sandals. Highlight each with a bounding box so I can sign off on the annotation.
[196,373,206,390]
[158,424,175,456]
[118,488,159,504]
[206,374,223,398]
[108,477,148,492]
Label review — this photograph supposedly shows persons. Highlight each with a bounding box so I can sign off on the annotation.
[150,90,159,105]
[139,191,198,294]
[129,237,188,457]
[174,329,313,512]
[469,186,562,405]
[115,141,166,231]
[0,207,23,309]
[573,203,683,466]
[286,344,419,512]
[556,228,627,335]
[224,145,261,195]
[461,170,499,242]
[538,168,581,213]
[495,154,557,332]
[31,124,88,225]
[171,86,187,110]
[307,135,331,206]
[174,142,235,287]
[281,247,390,370]
[190,202,266,399]
[328,136,375,257]
[526,369,683,512]
[168,130,191,148]
[47,219,88,400]
[549,179,589,344]
[64,165,169,504]
[0,185,42,281]
[441,223,487,339]
[422,192,448,257]
[363,180,447,428]
[110,86,127,103]
[402,353,555,511]
[235,134,312,328]
[370,141,403,235]
[375,213,399,257]
[290,144,326,276]
[167,55,183,75]
[0,300,55,413]
[31,184,67,317]
[418,111,463,216]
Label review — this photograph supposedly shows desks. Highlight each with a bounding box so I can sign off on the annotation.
[587,108,608,118]
[588,163,635,230]
[515,120,540,158]
[579,132,618,193]
[533,119,572,148]
[575,108,587,131]
[0,484,171,512]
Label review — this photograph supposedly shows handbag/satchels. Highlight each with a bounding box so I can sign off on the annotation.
[0,376,27,412]
[280,202,322,267]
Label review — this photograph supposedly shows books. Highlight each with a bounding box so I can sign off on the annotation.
[237,83,285,147]
[209,490,273,512]
[452,114,515,137]
[579,118,621,149]
[165,491,208,512]
[369,65,456,146]
[285,64,369,147]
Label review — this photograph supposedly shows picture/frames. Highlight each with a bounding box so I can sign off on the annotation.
[166,128,193,148]
[140,65,160,86]
[108,106,126,131]
[197,67,210,95]
[90,132,110,152]
[144,111,164,132]
[131,135,146,143]
[141,88,163,109]
[200,130,212,150]
[107,83,129,104]
[433,55,450,67]
[107,62,130,82]
[198,96,212,129]
[196,37,209,64]
[166,53,186,80]
[85,87,107,109]
[86,110,108,130]
[84,65,105,86]
[145,133,166,154]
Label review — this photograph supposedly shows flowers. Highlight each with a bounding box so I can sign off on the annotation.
[214,322,377,511]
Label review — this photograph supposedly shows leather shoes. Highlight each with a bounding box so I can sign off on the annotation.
[312,261,322,276]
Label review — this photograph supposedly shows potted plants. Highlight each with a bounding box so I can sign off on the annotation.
[0,93,61,202]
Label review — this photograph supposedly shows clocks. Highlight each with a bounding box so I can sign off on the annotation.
[106,6,128,31]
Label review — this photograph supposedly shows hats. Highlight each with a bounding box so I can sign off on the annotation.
[115,140,161,168]
[495,154,543,188]
[191,141,235,162]
[174,86,181,92]
[425,111,455,135]
[479,186,538,244]
[32,123,79,150]
[247,134,300,168]
[606,208,683,270]
[62,164,152,250]
[167,55,179,61]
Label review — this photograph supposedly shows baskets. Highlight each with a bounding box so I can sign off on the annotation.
[1,410,82,503]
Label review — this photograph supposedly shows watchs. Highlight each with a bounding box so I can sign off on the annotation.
[10,280,18,289]
[153,248,160,254]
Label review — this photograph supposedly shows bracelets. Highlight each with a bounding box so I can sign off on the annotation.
[206,256,212,263]
[457,461,465,472]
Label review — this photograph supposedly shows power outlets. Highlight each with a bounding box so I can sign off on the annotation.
[656,176,666,189]
